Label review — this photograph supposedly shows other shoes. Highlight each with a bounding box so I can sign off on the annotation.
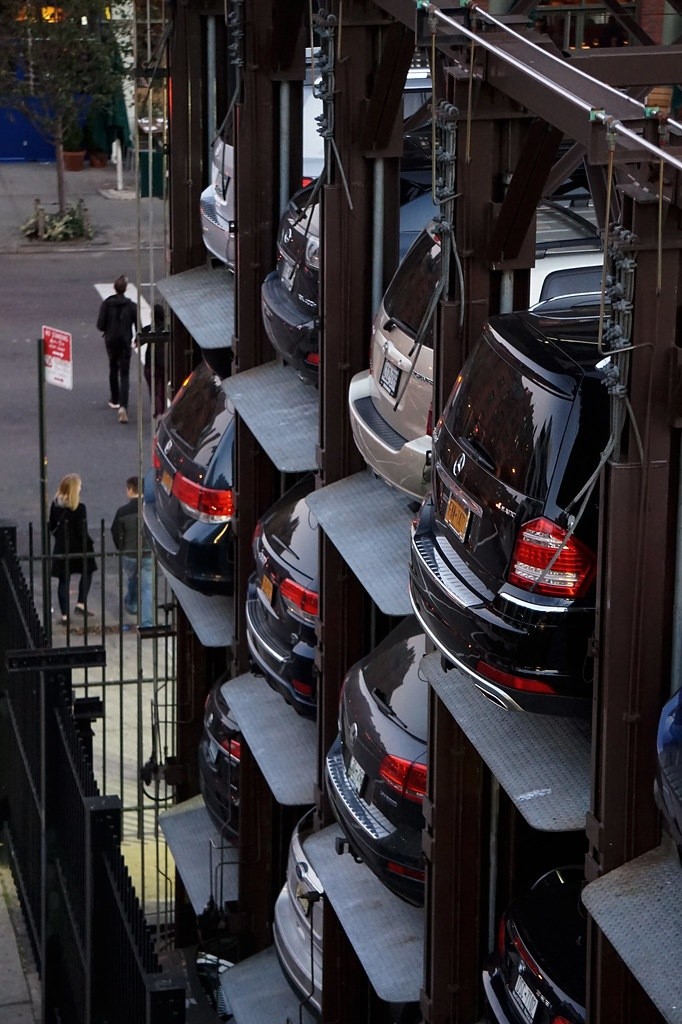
[125,595,138,615]
[59,616,68,625]
[74,607,94,617]
[107,402,120,409]
[118,406,128,423]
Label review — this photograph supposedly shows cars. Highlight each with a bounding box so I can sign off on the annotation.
[272,803,328,1017]
[199,677,239,843]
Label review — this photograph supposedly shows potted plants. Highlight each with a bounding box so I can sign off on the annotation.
[63,120,88,171]
[83,95,111,168]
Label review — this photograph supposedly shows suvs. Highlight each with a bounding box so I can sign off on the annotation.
[404,286,616,719]
[242,471,389,721]
[479,866,667,1024]
[349,195,600,504]
[198,46,444,373]
[139,366,304,600]
[319,617,586,912]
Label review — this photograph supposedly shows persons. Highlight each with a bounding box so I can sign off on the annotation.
[110,476,154,628]
[96,275,165,422]
[49,473,100,625]
